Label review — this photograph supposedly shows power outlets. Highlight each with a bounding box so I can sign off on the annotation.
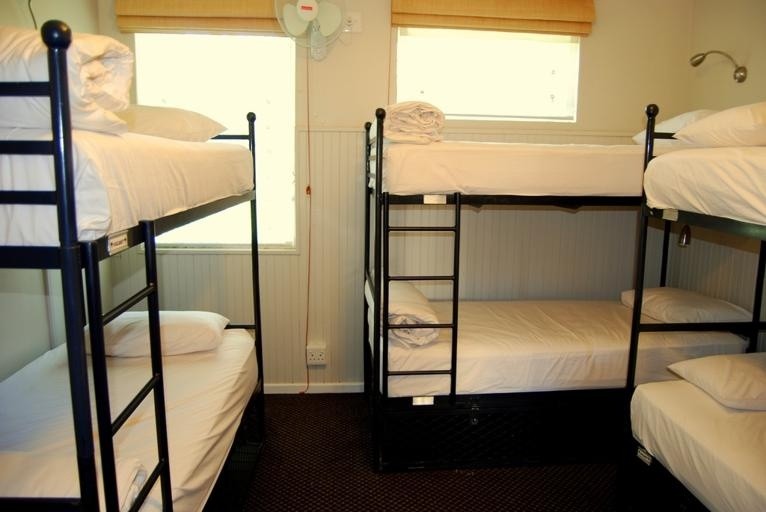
[307,345,327,366]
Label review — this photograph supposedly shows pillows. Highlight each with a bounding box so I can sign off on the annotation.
[84,311,230,357]
[631,109,722,146]
[621,287,752,323]
[667,352,766,411]
[114,106,226,142]
[672,103,765,147]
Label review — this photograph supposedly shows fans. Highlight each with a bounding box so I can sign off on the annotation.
[274,0,352,394]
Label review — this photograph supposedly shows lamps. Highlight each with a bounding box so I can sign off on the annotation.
[678,223,692,247]
[690,50,747,83]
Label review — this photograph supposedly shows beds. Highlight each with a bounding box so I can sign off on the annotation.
[364,108,766,434]
[621,104,766,512]
[0,20,263,512]
